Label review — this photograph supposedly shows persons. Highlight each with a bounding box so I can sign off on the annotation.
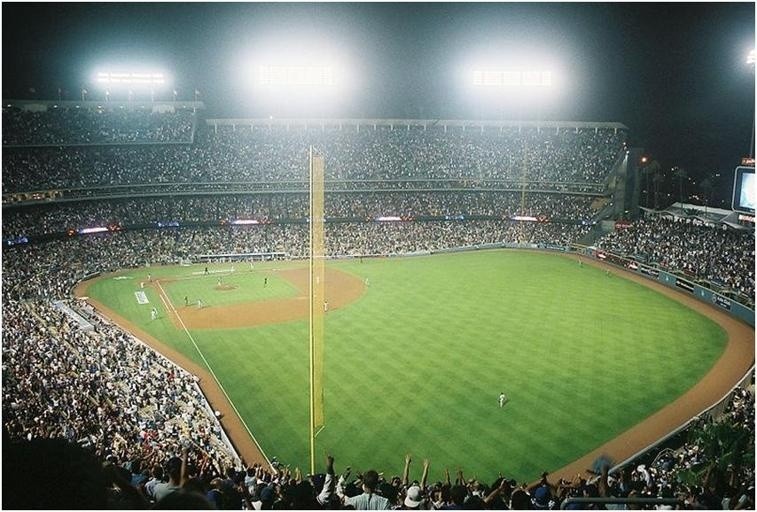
[238,449,753,509]
[630,213,754,313]
[496,392,506,408]
[323,301,329,311]
[1,263,238,509]
[655,370,755,449]
[4,104,630,263]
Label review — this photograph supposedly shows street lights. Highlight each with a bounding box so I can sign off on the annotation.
[641,157,648,207]
[745,50,755,160]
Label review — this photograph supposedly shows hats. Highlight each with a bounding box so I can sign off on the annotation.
[534,487,551,502]
[586,458,609,475]
[403,485,422,508]
